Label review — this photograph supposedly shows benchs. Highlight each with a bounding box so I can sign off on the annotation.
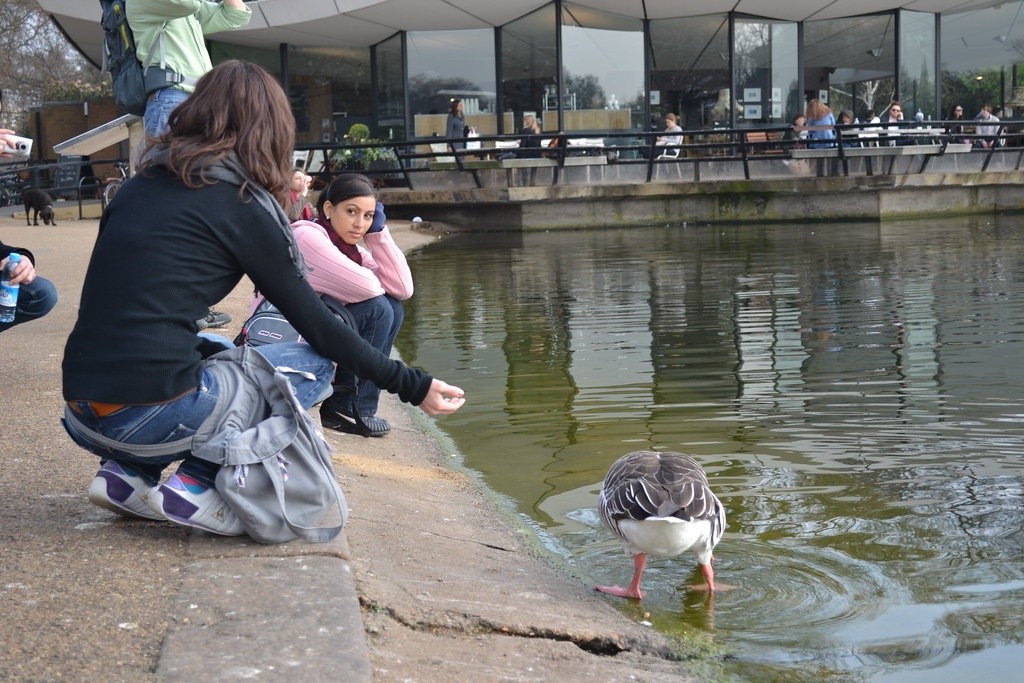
[791,144,973,174]
[747,130,788,154]
[428,156,609,183]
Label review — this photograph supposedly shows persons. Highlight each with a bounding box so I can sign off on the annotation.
[515,115,542,186]
[446,99,477,162]
[124,0,253,328]
[835,101,914,147]
[0,89,58,333]
[944,104,968,144]
[975,102,1005,147]
[289,168,317,222]
[806,99,835,149]
[781,113,806,152]
[61,59,465,536]
[247,172,414,435]
[653,113,683,158]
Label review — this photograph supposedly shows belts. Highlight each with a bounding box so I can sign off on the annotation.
[69,399,126,415]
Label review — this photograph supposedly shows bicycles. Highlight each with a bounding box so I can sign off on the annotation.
[103,162,130,207]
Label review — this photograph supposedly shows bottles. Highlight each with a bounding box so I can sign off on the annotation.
[460,98,478,114]
[927,114,931,129]
[571,92,577,110]
[853,118,860,132]
[916,108,924,129]
[0,253,20,323]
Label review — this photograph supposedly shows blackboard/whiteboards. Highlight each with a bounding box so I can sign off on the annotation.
[55,154,99,202]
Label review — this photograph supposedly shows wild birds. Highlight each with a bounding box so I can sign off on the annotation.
[591,450,726,600]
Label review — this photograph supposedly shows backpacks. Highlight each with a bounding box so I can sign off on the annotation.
[99,0,201,117]
[190,346,348,545]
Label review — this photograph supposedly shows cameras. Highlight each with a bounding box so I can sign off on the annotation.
[2,134,33,156]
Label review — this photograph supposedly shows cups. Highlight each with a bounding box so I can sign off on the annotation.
[603,94,619,110]
[302,175,312,184]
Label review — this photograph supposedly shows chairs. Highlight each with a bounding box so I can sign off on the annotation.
[653,137,684,181]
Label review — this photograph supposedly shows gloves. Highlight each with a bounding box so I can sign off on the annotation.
[369,201,386,232]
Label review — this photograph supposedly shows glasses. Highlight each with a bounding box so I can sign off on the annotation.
[955,108,963,111]
[891,109,899,112]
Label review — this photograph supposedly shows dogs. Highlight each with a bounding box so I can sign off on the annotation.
[23,188,57,226]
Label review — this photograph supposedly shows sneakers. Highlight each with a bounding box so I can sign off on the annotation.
[320,400,390,437]
[204,307,231,326]
[151,474,247,537]
[89,460,167,522]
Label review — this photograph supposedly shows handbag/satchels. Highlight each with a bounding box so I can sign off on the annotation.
[233,291,359,382]
[465,129,481,150]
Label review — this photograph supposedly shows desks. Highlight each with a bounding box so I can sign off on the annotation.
[842,128,946,141]
